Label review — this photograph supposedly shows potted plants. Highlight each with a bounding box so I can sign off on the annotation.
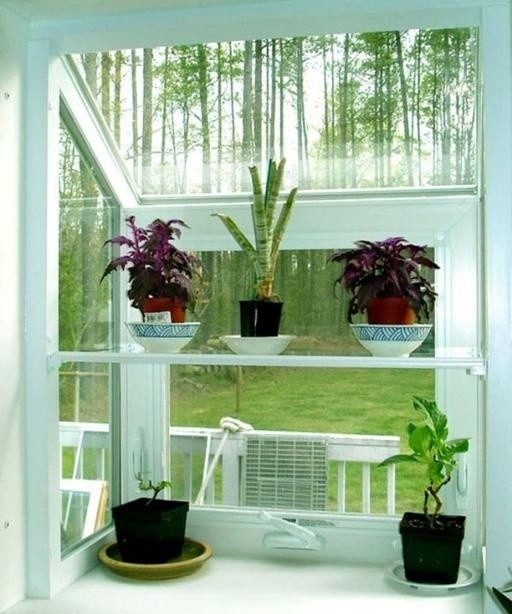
[214,153,304,334]
[108,473,194,562]
[96,217,207,322]
[378,395,472,585]
[332,236,440,324]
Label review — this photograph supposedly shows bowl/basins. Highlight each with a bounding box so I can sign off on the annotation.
[350,324,431,357]
[220,333,296,356]
[124,322,201,354]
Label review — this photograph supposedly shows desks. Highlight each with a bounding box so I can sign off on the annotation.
[9,546,506,613]
